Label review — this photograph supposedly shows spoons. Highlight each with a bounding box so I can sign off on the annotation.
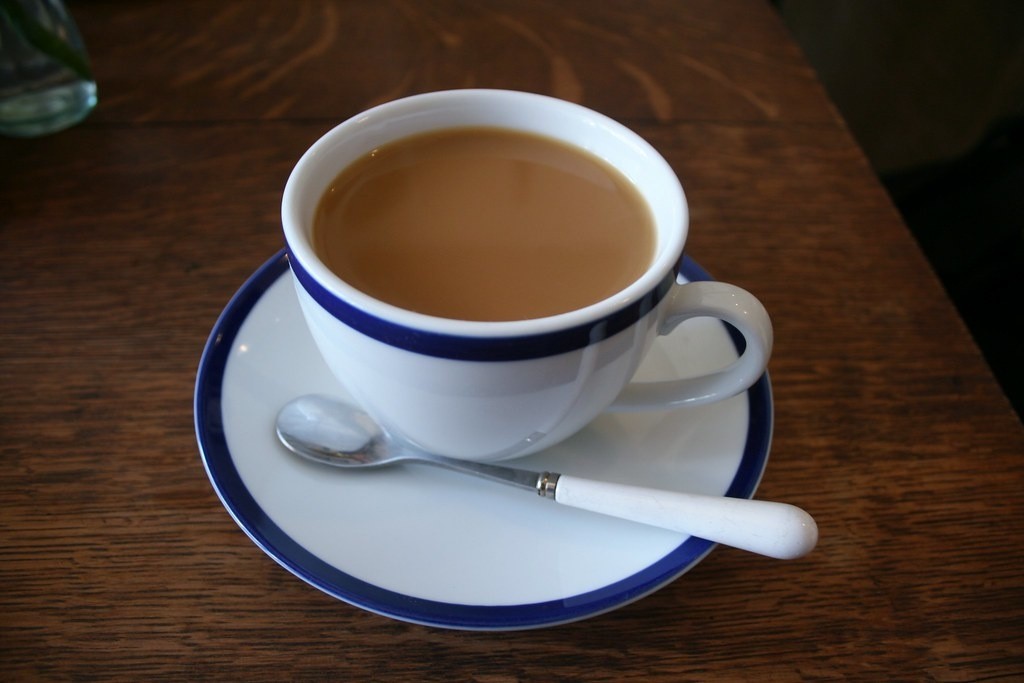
[274,394,819,562]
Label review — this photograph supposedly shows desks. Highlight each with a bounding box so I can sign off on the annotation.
[1,0,1022,681]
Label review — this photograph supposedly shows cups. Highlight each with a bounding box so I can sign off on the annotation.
[0,0,98,137]
[278,88,775,465]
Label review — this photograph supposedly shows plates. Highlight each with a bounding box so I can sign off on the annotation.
[192,243,774,632]
